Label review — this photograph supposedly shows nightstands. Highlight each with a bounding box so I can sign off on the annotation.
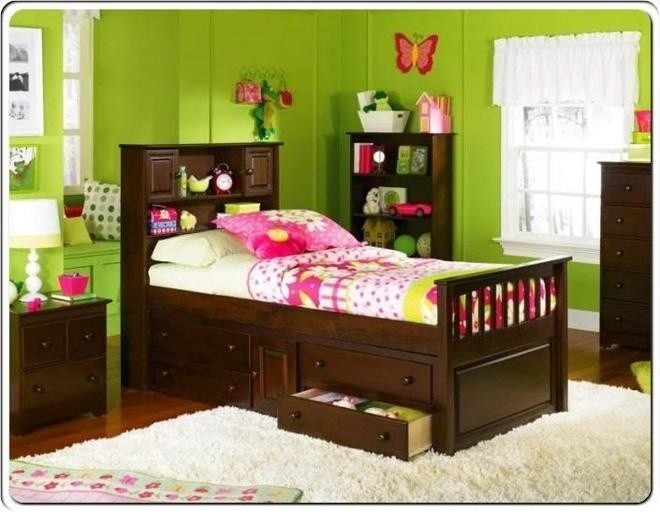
[11,292,108,439]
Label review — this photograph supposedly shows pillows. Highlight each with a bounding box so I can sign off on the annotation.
[82,173,120,244]
[150,226,242,267]
[213,200,365,261]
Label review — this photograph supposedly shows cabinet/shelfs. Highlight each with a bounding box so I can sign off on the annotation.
[115,135,285,234]
[346,128,452,261]
[146,312,251,410]
[277,332,436,461]
[595,156,651,353]
[65,245,121,338]
[247,327,298,419]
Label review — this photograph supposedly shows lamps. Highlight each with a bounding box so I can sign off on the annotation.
[7,197,65,306]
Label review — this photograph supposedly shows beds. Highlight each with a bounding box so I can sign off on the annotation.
[116,244,575,464]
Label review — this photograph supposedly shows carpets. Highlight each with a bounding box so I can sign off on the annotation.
[22,372,652,502]
[8,458,302,500]
[627,359,652,395]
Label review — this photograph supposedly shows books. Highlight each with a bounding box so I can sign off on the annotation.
[50,293,96,302]
[353,142,370,175]
[310,389,428,425]
[359,144,364,174]
[364,145,385,174]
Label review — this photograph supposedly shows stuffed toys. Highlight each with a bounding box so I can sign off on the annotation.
[362,90,394,115]
[179,209,199,232]
[362,186,381,215]
